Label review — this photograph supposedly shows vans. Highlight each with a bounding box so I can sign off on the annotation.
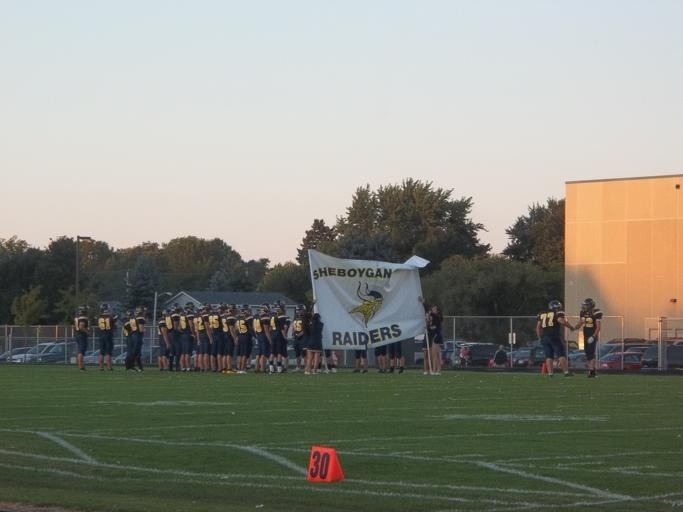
[0,339,161,366]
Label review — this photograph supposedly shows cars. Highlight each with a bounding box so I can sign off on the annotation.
[414,336,683,369]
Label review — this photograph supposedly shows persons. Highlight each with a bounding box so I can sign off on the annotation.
[124,306,147,375]
[492,344,510,371]
[575,298,603,379]
[535,301,575,378]
[157,300,405,375]
[94,303,120,371]
[71,303,91,373]
[420,303,444,376]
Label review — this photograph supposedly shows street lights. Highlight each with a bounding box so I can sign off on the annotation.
[153,292,173,345]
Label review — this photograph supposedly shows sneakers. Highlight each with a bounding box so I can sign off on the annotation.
[76,364,407,374]
[422,370,441,376]
[547,368,598,379]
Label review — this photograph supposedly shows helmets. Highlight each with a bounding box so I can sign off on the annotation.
[580,298,596,312]
[549,299,562,311]
[76,304,89,315]
[125,309,135,320]
[136,306,146,314]
[161,298,285,320]
[99,303,113,314]
[295,303,306,313]
